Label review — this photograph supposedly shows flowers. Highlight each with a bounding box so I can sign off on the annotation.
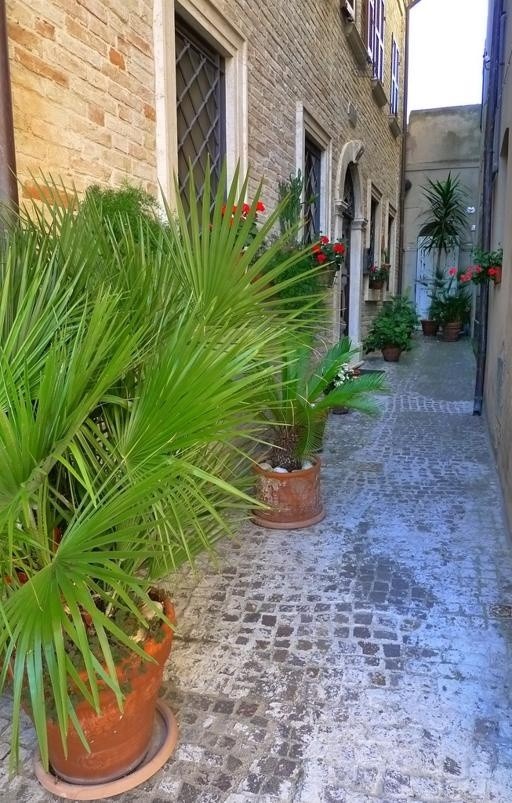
[449,247,505,285]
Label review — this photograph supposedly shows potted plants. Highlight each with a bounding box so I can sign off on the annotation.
[1,152,390,803]
[413,264,448,337]
[363,309,411,362]
[440,291,474,343]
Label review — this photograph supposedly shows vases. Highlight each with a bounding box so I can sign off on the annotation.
[494,265,501,284]
